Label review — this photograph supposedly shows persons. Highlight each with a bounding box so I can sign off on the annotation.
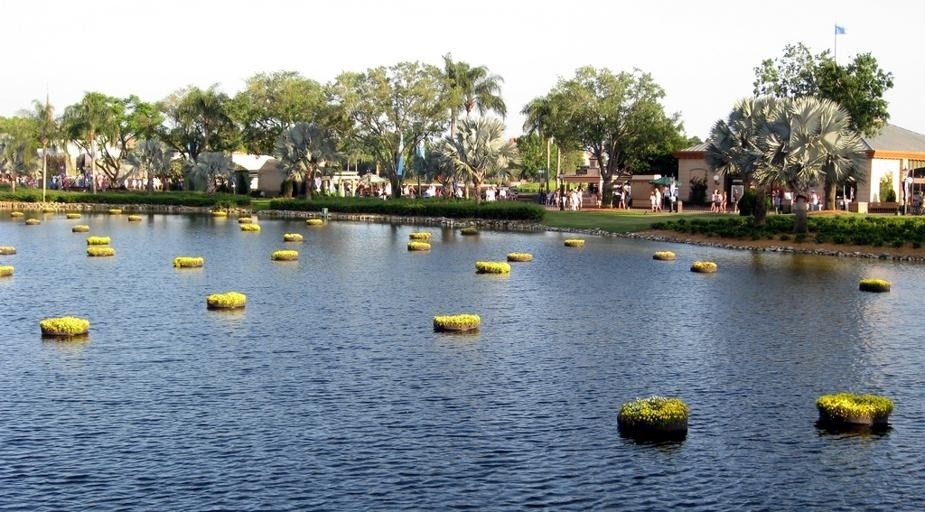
[342,179,458,199]
[809,190,819,206]
[484,184,663,213]
[0,172,185,193]
[710,188,741,213]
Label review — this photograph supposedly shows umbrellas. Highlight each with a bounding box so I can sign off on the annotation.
[647,175,682,209]
